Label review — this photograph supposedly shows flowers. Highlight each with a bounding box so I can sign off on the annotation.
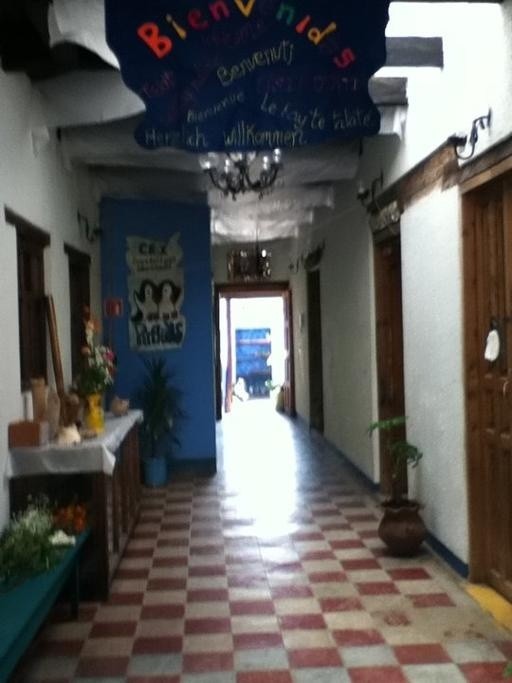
[72,319,116,398]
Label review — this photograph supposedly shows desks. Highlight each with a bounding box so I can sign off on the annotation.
[0,524,93,683]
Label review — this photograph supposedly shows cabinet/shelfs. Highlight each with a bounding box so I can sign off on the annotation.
[5,409,145,601]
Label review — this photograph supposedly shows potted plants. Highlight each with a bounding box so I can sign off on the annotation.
[136,354,183,485]
[362,418,430,559]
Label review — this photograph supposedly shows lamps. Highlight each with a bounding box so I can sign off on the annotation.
[356,169,383,208]
[444,106,491,160]
[288,252,306,275]
[228,241,272,280]
[197,147,284,201]
[76,209,103,243]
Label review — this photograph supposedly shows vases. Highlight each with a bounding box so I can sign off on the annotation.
[85,389,105,436]
